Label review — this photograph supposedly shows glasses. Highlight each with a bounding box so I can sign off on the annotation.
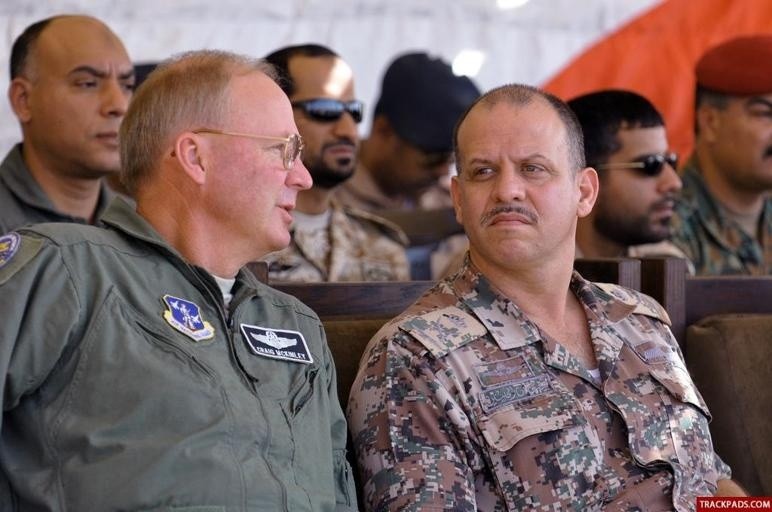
[587,152,680,176]
[169,123,304,170]
[288,97,363,125]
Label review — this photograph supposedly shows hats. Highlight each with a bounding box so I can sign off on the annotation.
[381,50,481,151]
[695,34,771,93]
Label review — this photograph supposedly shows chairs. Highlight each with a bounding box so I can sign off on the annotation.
[364,206,465,280]
[247,257,642,414]
[640,257,772,497]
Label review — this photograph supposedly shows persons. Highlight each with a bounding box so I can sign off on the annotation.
[335,50,482,283]
[0,13,136,238]
[665,32,772,279]
[0,49,358,512]
[345,79,750,512]
[565,86,698,277]
[254,41,412,285]
